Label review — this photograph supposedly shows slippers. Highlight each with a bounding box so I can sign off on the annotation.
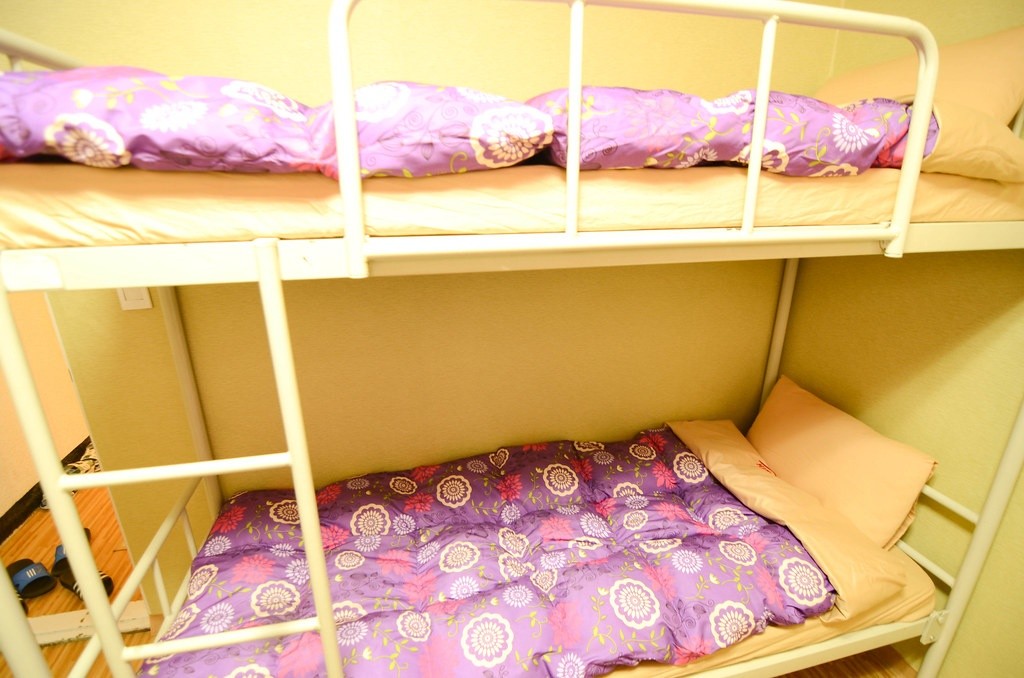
[52,528,91,577]
[59,570,114,601]
[39,442,101,509]
[6,558,57,615]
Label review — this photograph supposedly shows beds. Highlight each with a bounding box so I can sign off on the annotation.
[0,1,1024,678]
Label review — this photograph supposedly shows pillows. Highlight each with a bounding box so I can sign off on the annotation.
[813,23,1024,127]
[747,372,936,550]
